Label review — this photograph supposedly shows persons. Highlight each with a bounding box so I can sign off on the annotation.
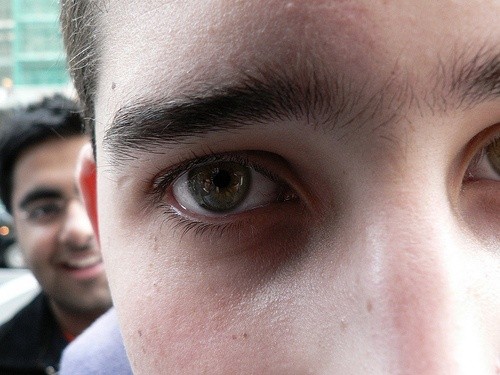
[0,94,115,375]
[49,0,499,375]
